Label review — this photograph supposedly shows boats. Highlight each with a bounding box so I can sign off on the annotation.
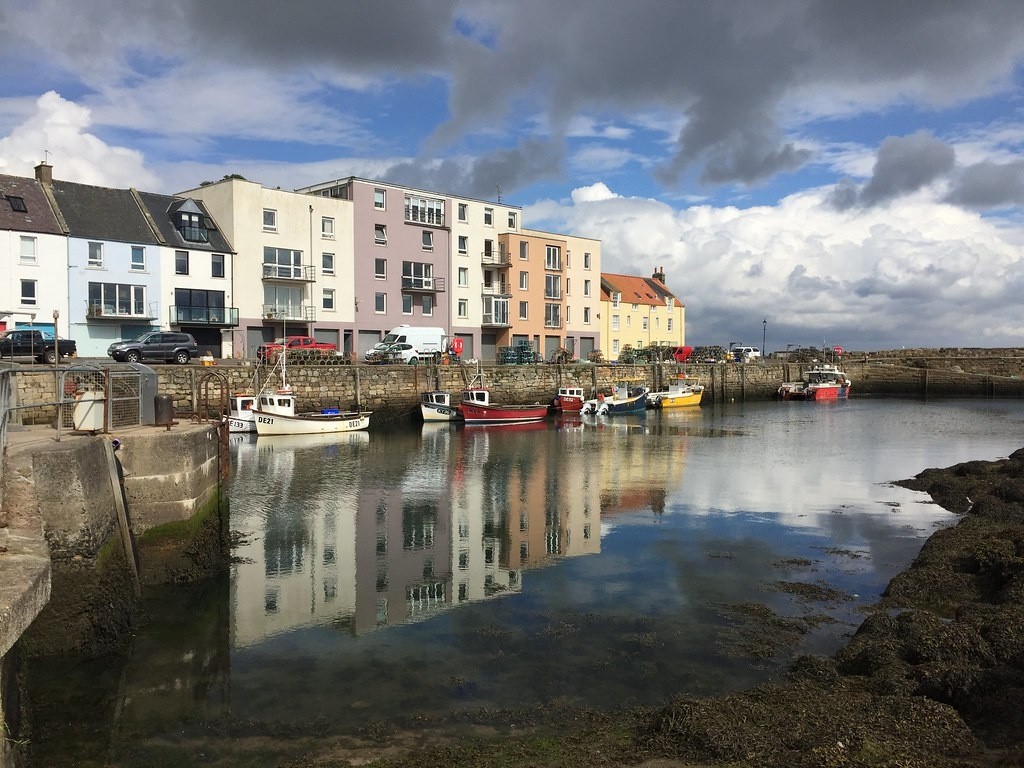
[646,377,704,408]
[550,388,650,415]
[221,305,373,436]
[778,344,852,401]
[419,361,551,423]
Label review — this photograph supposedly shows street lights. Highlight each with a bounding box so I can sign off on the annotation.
[762,321,767,361]
[52,310,59,368]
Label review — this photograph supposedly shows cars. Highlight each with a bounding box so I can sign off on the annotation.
[369,342,419,365]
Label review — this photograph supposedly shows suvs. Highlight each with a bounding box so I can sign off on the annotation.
[107,331,200,365]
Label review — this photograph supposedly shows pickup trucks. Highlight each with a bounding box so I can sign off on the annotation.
[257,335,337,361]
[0,330,76,364]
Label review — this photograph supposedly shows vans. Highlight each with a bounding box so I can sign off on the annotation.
[365,324,447,362]
[664,347,692,362]
[728,346,761,363]
